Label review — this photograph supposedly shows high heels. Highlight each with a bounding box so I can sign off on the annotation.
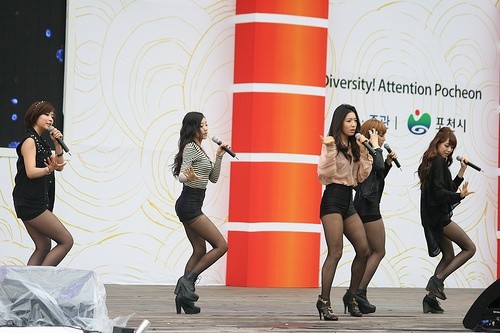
[347,290,376,313]
[425,275,447,300]
[342,289,364,317]
[173,272,200,301]
[422,294,445,314]
[175,295,201,314]
[315,295,339,321]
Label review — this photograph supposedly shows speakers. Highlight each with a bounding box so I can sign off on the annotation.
[462,279,500,329]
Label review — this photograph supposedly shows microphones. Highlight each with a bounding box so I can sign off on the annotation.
[48,126,71,157]
[457,156,485,173]
[384,144,402,170]
[355,133,377,158]
[211,137,239,161]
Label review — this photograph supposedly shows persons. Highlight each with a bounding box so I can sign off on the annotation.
[173,112,231,314]
[418,127,476,314]
[353,118,397,313]
[316,104,373,322]
[13,101,74,267]
